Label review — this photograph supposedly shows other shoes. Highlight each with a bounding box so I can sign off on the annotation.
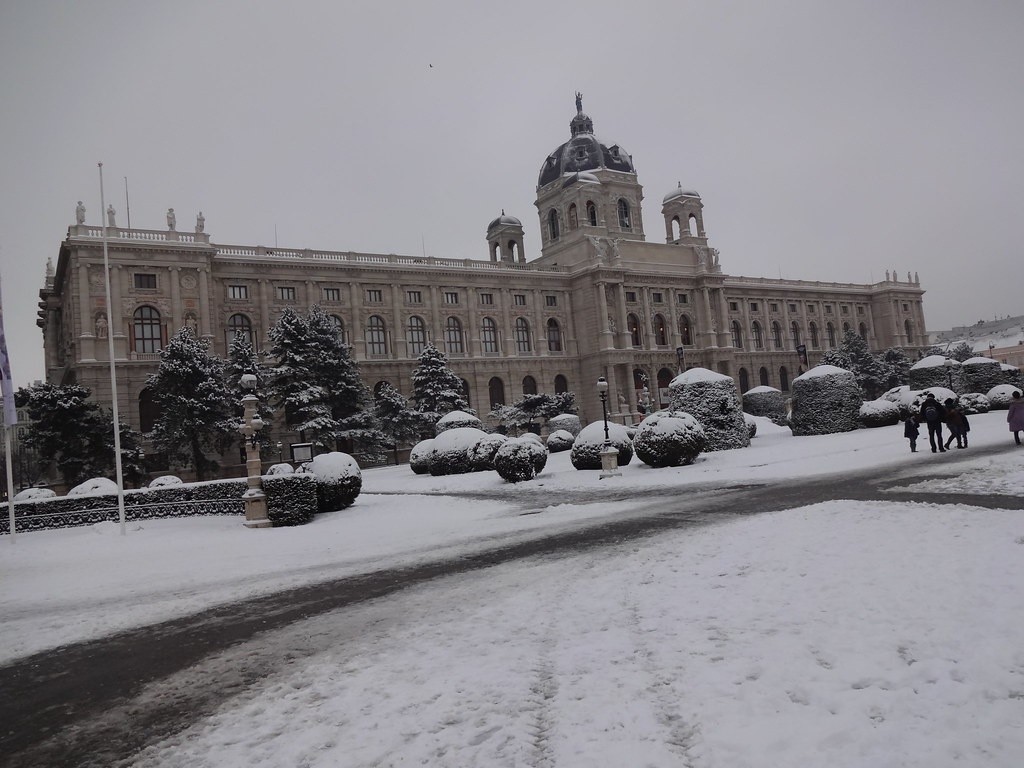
[958,446,964,449]
[964,446,967,448]
[944,446,950,450]
[1017,442,1021,445]
[940,450,946,452]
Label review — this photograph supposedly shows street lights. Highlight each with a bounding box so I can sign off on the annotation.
[239,368,274,528]
[599,377,622,480]
[944,357,953,391]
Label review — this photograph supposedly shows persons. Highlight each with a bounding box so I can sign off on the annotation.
[167,207,176,231]
[76,200,86,224]
[1007,390,1024,446]
[915,272,919,283]
[893,270,897,281]
[908,271,913,282]
[886,269,890,281]
[899,392,970,454]
[195,211,206,232]
[106,204,116,226]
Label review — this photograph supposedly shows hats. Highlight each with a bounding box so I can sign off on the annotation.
[1012,391,1020,399]
[944,398,953,404]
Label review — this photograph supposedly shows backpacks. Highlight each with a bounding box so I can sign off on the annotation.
[924,406,937,421]
